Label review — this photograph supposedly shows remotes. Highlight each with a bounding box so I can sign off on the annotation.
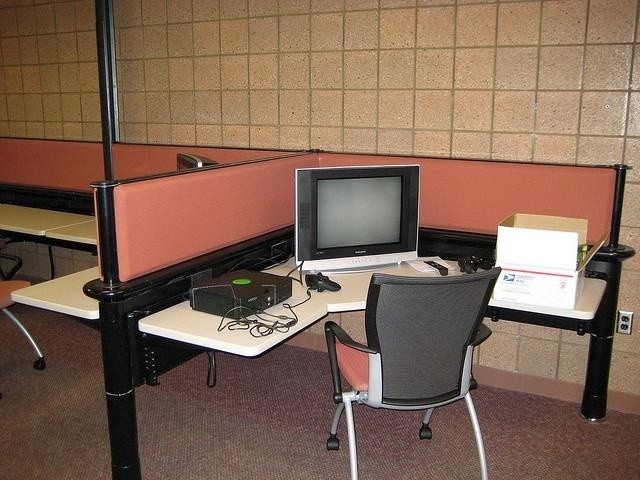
[423,260,448,277]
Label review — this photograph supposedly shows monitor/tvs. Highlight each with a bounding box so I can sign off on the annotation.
[176,152,219,170]
[293,163,421,275]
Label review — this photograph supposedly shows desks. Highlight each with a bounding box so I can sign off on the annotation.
[0,135,635,479]
